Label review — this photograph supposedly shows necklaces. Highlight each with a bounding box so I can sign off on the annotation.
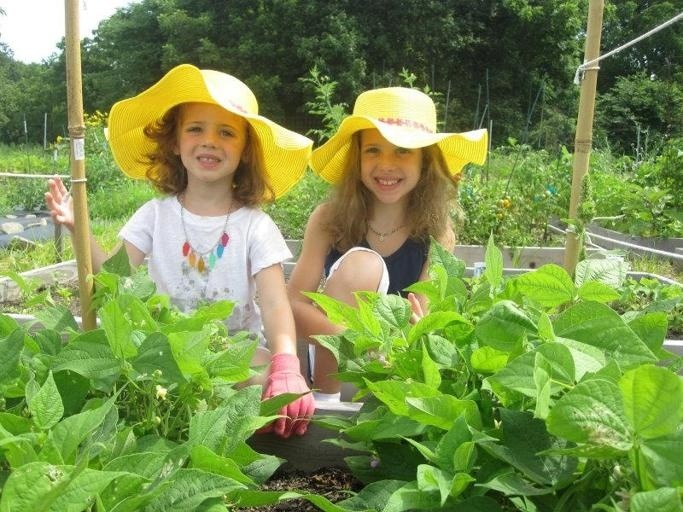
[366,221,411,241]
[180,195,234,272]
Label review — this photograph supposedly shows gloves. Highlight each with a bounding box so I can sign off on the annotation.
[256,352,315,438]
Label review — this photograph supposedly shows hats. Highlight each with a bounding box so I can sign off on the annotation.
[309,87,488,185]
[108,64,315,199]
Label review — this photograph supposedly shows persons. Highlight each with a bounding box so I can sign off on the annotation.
[43,63,315,439]
[285,87,488,405]
